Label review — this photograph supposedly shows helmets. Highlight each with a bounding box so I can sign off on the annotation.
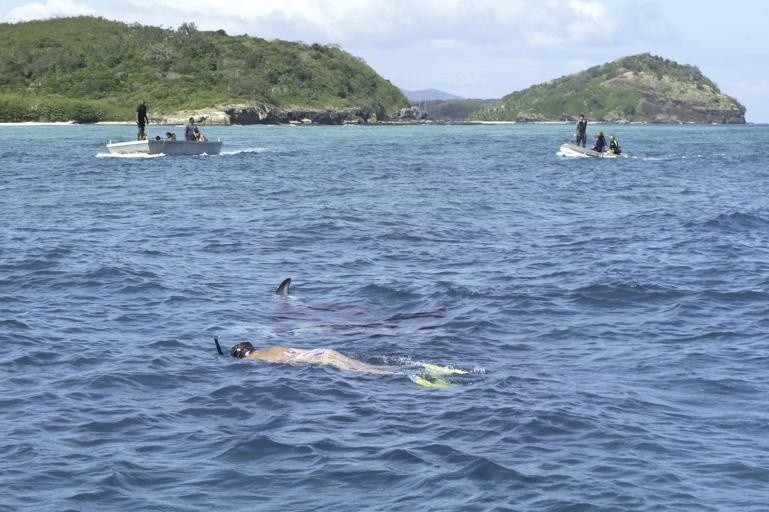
[230,342,254,359]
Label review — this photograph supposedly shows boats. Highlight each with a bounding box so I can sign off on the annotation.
[106,135,223,158]
[558,143,629,161]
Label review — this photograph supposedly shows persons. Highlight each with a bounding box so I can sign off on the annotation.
[574,114,587,148]
[142,127,176,142]
[591,131,621,156]
[183,117,208,141]
[230,341,470,396]
[136,98,149,140]
